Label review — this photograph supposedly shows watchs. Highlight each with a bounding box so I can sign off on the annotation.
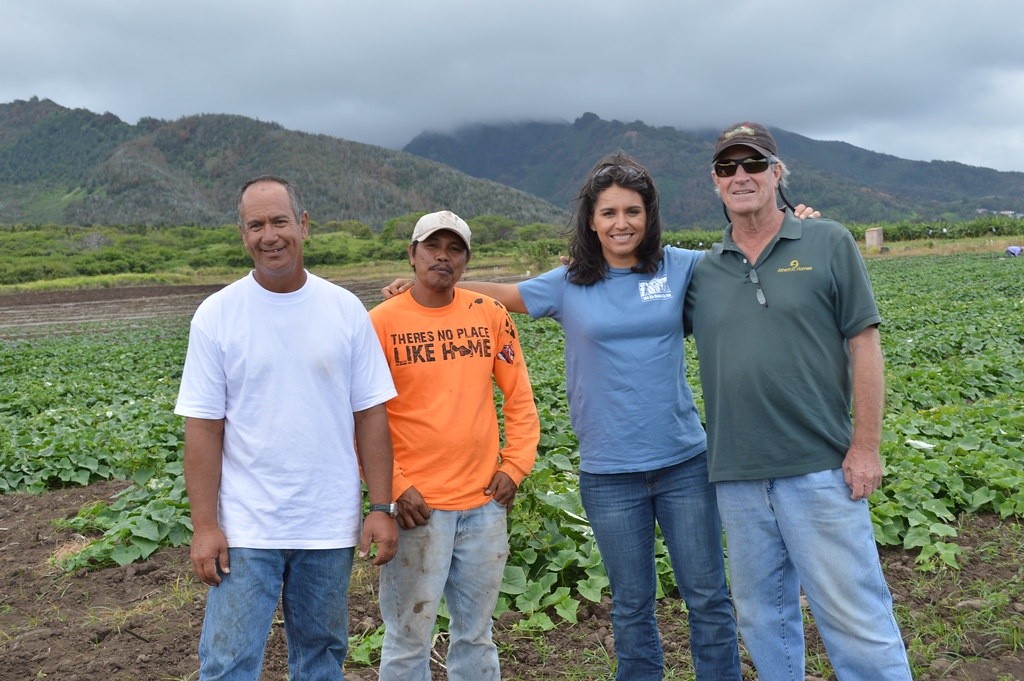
[368,501,399,519]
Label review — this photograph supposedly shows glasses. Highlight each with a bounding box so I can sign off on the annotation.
[749,267,768,308]
[714,155,776,177]
[593,164,647,181]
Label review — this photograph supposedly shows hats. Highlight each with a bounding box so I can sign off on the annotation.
[713,122,778,161]
[411,210,471,243]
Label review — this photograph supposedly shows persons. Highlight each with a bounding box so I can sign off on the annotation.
[171,173,401,681]
[1004,245,1024,257]
[354,209,539,681]
[557,122,915,681]
[380,149,824,681]
